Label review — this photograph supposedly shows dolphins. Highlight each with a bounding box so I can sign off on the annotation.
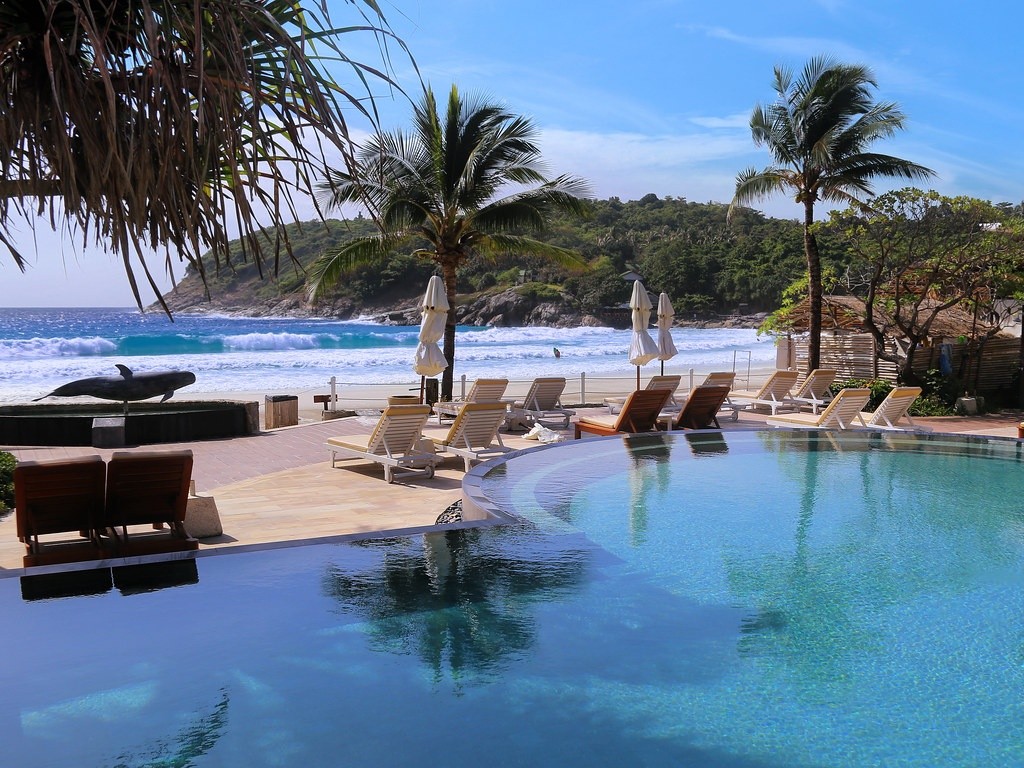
[33,364,197,404]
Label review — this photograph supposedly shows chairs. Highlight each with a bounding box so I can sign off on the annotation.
[422,402,519,472]
[725,370,809,416]
[572,389,672,440]
[100,449,199,554]
[671,371,742,423]
[765,388,873,429]
[506,377,577,428]
[13,454,108,567]
[757,369,837,414]
[431,378,509,426]
[821,386,934,433]
[654,385,731,431]
[603,375,682,416]
[323,404,436,483]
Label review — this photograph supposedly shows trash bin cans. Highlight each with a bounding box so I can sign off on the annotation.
[1017,420,1024,438]
[265,394,298,430]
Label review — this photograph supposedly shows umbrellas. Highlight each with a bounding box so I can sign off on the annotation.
[628,279,660,392]
[656,292,679,377]
[413,275,461,411]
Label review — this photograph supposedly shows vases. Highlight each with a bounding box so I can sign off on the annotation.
[388,395,420,405]
